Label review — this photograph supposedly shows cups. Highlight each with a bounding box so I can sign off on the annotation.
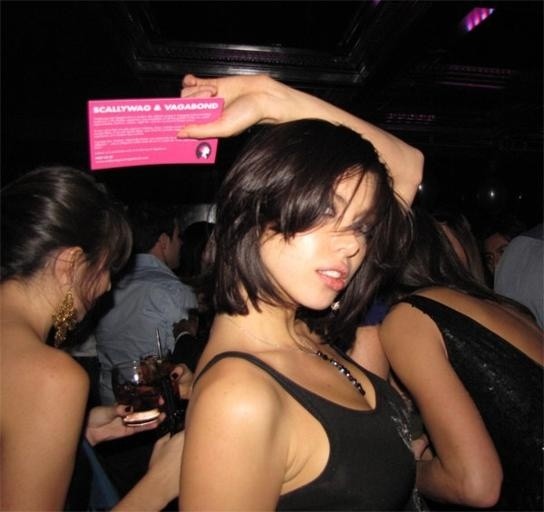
[139,349,180,415]
[110,359,165,427]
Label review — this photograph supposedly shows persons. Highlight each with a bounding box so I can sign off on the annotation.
[173,71,432,511]
[378,200,543,510]
[0,164,186,511]
